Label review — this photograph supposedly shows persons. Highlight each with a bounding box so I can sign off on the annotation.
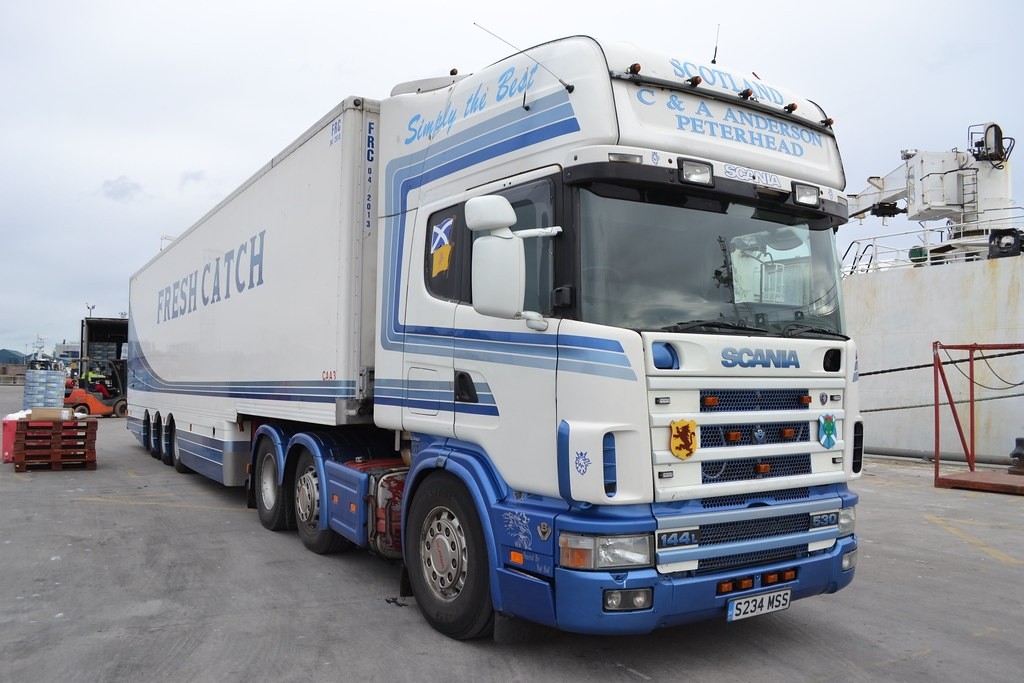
[81,367,115,399]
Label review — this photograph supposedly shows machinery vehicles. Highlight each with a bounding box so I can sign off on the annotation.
[44,355,126,421]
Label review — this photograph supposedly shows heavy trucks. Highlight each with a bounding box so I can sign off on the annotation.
[119,38,874,649]
